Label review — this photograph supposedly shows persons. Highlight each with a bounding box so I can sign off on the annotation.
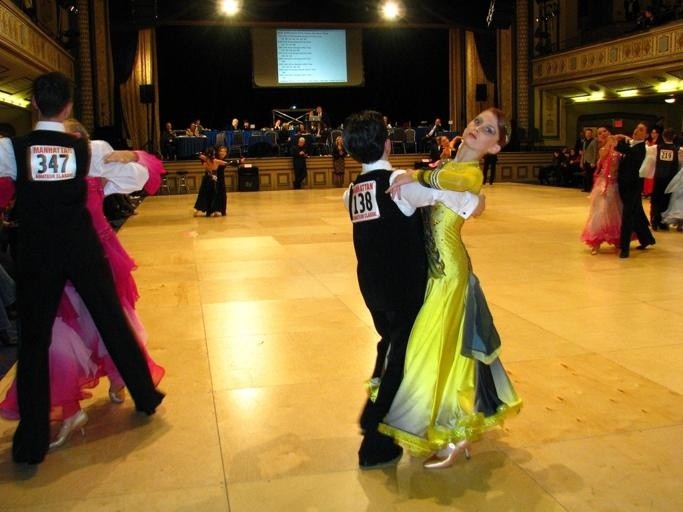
[340,108,524,469]
[550,122,682,259]
[161,107,347,217]
[1,72,167,479]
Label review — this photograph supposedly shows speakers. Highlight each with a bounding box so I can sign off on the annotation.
[237,166,259,191]
[140,84,155,103]
[475,83,488,102]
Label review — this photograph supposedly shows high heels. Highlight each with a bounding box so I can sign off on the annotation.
[48,410,89,448]
[591,247,600,255]
[423,439,471,470]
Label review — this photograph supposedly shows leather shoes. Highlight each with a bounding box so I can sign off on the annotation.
[635,237,655,250]
[359,445,404,470]
[619,249,630,258]
